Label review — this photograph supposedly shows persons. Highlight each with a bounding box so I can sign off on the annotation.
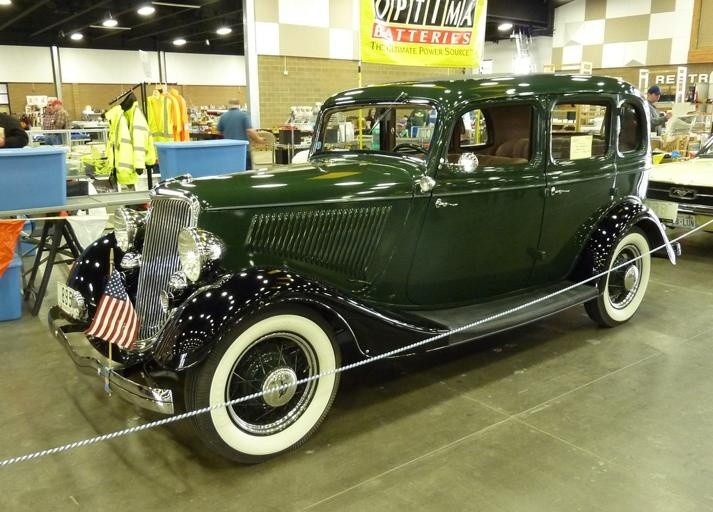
[0,111,29,149]
[217,96,266,170]
[41,103,56,145]
[363,104,477,145]
[645,84,672,131]
[51,99,68,144]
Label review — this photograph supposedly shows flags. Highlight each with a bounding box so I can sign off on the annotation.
[83,264,141,352]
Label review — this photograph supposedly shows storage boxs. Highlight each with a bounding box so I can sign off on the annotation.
[1,139,250,213]
[1,253,23,322]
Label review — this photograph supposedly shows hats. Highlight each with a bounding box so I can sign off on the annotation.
[49,100,63,107]
[648,85,662,95]
